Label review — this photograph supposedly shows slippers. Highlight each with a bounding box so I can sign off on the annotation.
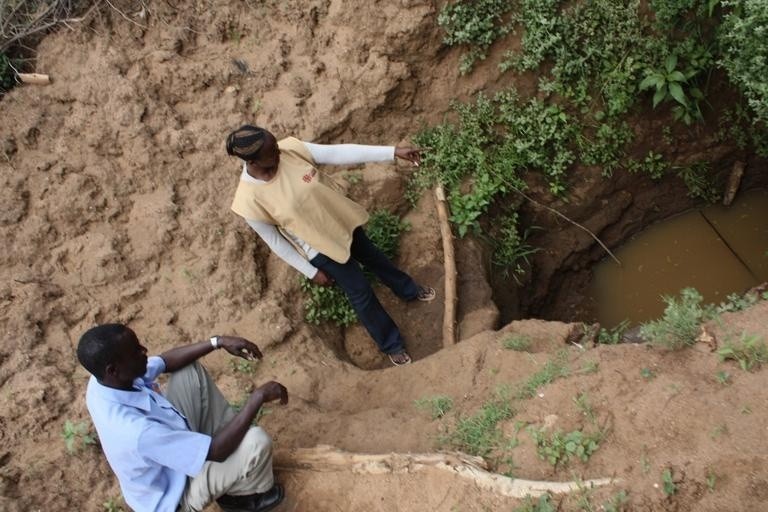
[416,284,436,301]
[386,350,412,366]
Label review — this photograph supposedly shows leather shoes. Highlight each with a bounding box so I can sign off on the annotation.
[216,484,283,512]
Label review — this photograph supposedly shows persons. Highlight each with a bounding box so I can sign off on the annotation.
[76,323,289,512]
[226,124,436,367]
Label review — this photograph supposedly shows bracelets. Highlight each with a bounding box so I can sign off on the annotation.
[210,335,221,350]
